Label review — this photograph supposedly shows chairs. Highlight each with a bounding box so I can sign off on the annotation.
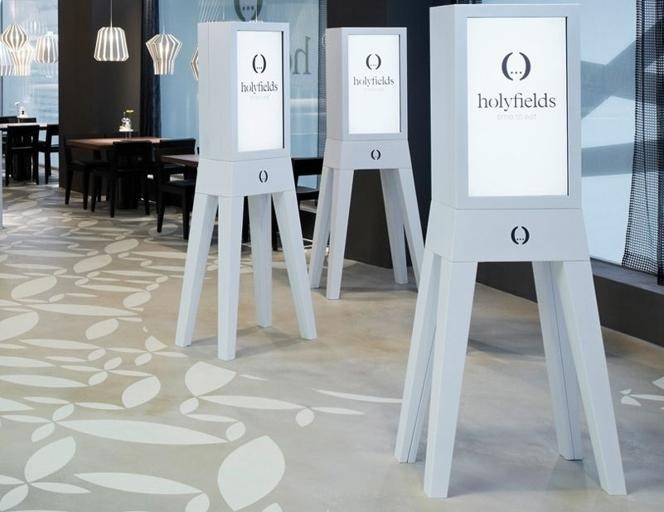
[290,156,323,213]
[108,137,199,242]
[1,115,58,186]
[63,128,138,209]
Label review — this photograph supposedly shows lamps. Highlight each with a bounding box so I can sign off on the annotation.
[0,0,59,76]
[192,0,227,80]
[145,0,182,74]
[93,1,129,62]
